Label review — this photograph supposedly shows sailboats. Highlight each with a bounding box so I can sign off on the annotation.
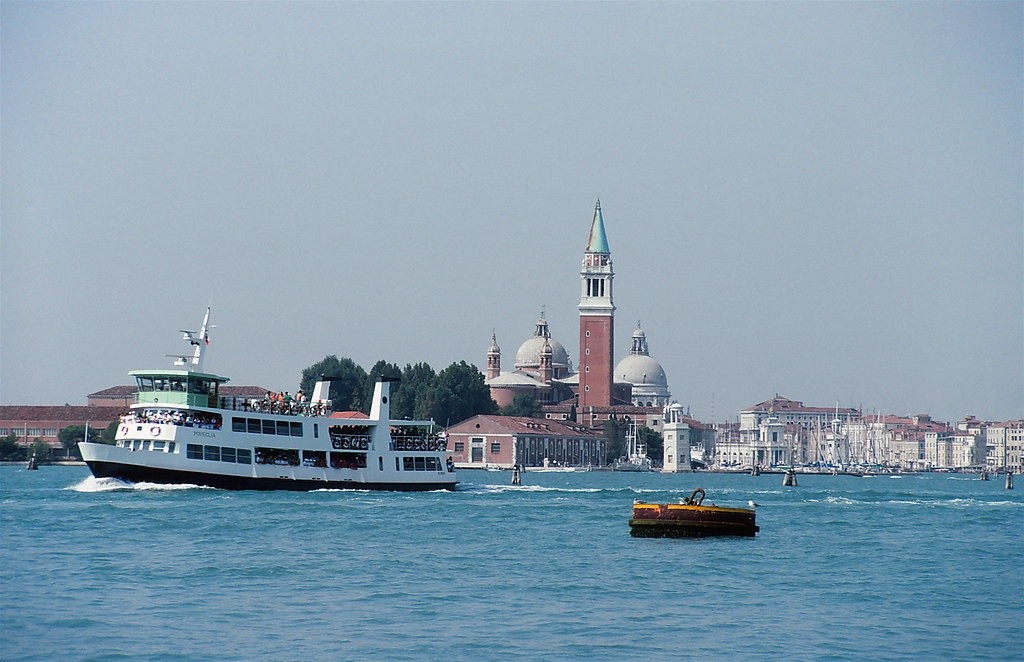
[687,401,923,478]
[612,414,654,472]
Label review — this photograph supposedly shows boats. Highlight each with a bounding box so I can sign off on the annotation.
[75,306,462,493]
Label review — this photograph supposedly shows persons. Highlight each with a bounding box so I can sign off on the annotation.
[121,391,450,473]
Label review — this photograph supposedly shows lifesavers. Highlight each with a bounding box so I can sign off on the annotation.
[333,436,342,449]
[280,403,289,414]
[121,426,128,436]
[436,439,446,449]
[351,438,360,448]
[261,402,270,412]
[360,438,368,449]
[317,408,326,417]
[341,437,350,448]
[150,426,161,436]
[310,408,316,415]
[251,401,260,412]
[406,438,412,450]
[419,438,427,450]
[301,404,310,414]
[291,402,300,410]
[428,439,434,449]
[271,402,280,413]
[414,438,420,450]
